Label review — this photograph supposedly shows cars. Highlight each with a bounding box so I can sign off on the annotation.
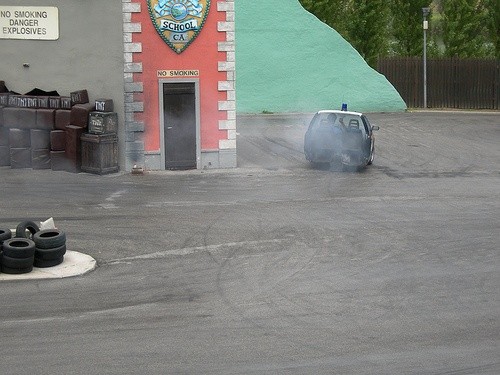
[304,102,381,171]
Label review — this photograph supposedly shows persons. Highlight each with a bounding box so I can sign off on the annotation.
[322,113,348,134]
